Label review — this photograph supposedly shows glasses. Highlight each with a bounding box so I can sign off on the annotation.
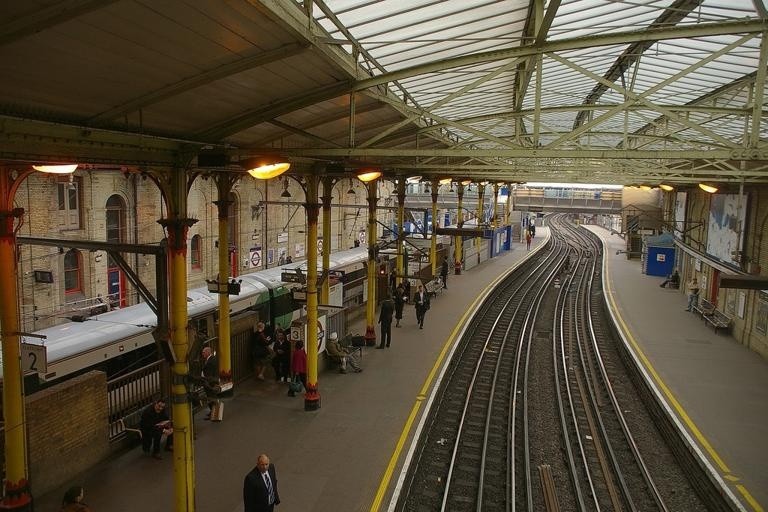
[461,175,471,185]
[697,178,722,193]
[439,170,452,185]
[639,180,654,190]
[244,137,291,182]
[659,179,678,192]
[480,178,489,185]
[355,154,381,183]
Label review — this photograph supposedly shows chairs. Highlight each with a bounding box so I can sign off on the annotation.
[374,323,425,350]
[255,373,289,386]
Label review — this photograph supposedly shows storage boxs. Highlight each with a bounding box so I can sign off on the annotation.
[120,399,159,444]
[691,296,716,317]
[423,279,444,300]
[702,308,732,334]
[325,330,364,368]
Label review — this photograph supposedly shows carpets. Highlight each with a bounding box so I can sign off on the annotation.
[264,473,275,504]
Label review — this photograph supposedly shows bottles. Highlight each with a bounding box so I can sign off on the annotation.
[330,332,338,343]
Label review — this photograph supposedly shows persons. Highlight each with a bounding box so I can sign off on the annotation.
[526,222,535,251]
[138,399,171,460]
[326,331,362,375]
[58,486,91,512]
[287,340,307,397]
[270,333,290,384]
[685,278,701,314]
[242,453,280,512]
[249,322,272,382]
[277,251,293,265]
[659,270,679,289]
[200,346,219,421]
[374,278,430,350]
[440,256,449,290]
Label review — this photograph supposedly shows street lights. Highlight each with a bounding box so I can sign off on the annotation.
[289,381,303,393]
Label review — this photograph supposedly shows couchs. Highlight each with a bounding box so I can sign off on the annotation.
[379,264,386,276]
